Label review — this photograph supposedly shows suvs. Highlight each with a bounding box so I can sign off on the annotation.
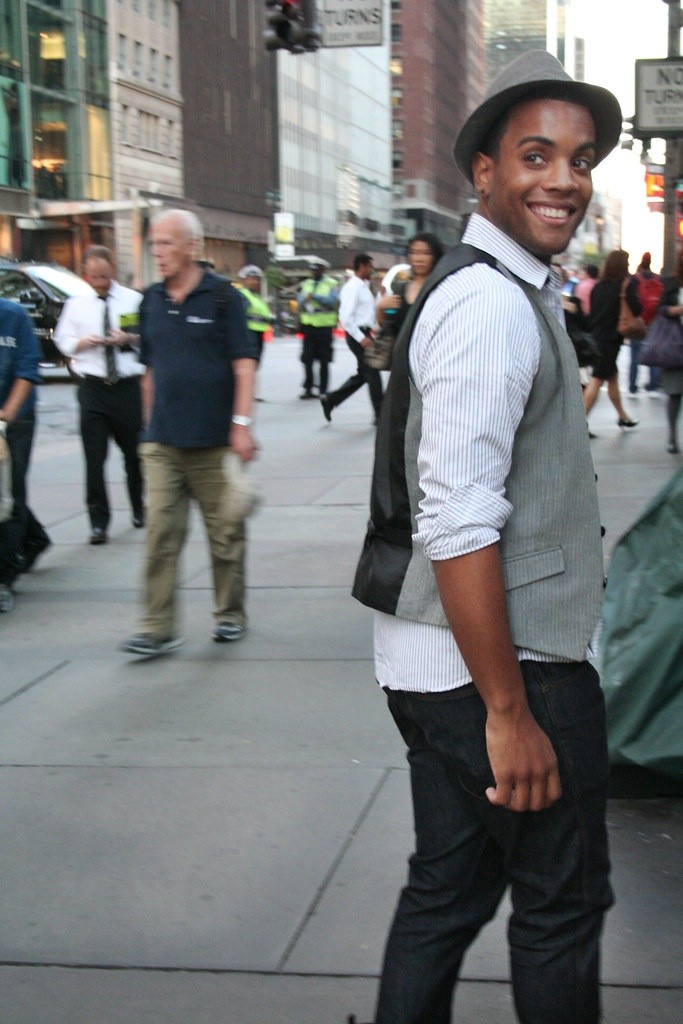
[0,261,98,384]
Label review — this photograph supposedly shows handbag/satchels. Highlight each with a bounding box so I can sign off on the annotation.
[366,318,394,369]
[573,321,611,368]
[638,311,683,368]
[618,278,646,339]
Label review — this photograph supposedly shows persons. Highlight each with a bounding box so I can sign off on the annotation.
[53,244,146,544]
[119,209,260,654]
[351,46,625,1024]
[0,296,49,613]
[229,232,683,453]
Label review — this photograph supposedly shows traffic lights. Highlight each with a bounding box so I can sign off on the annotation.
[261,0,321,53]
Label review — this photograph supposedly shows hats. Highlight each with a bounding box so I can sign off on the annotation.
[453,50,622,186]
[305,256,331,270]
[238,264,264,279]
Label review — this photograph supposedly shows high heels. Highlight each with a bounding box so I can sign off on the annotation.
[619,419,638,430]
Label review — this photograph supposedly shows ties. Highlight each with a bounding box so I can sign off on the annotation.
[99,294,117,385]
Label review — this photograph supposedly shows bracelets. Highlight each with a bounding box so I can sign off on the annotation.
[231,414,251,427]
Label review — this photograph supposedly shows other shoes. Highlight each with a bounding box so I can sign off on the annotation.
[90,526,105,543]
[134,515,144,526]
[16,535,49,575]
[667,439,678,454]
[321,396,334,422]
[300,389,312,398]
[319,390,326,398]
[0,584,15,612]
[629,383,637,394]
[645,380,659,391]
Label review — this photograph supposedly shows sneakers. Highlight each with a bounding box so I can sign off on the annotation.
[212,621,245,641]
[122,632,188,654]
